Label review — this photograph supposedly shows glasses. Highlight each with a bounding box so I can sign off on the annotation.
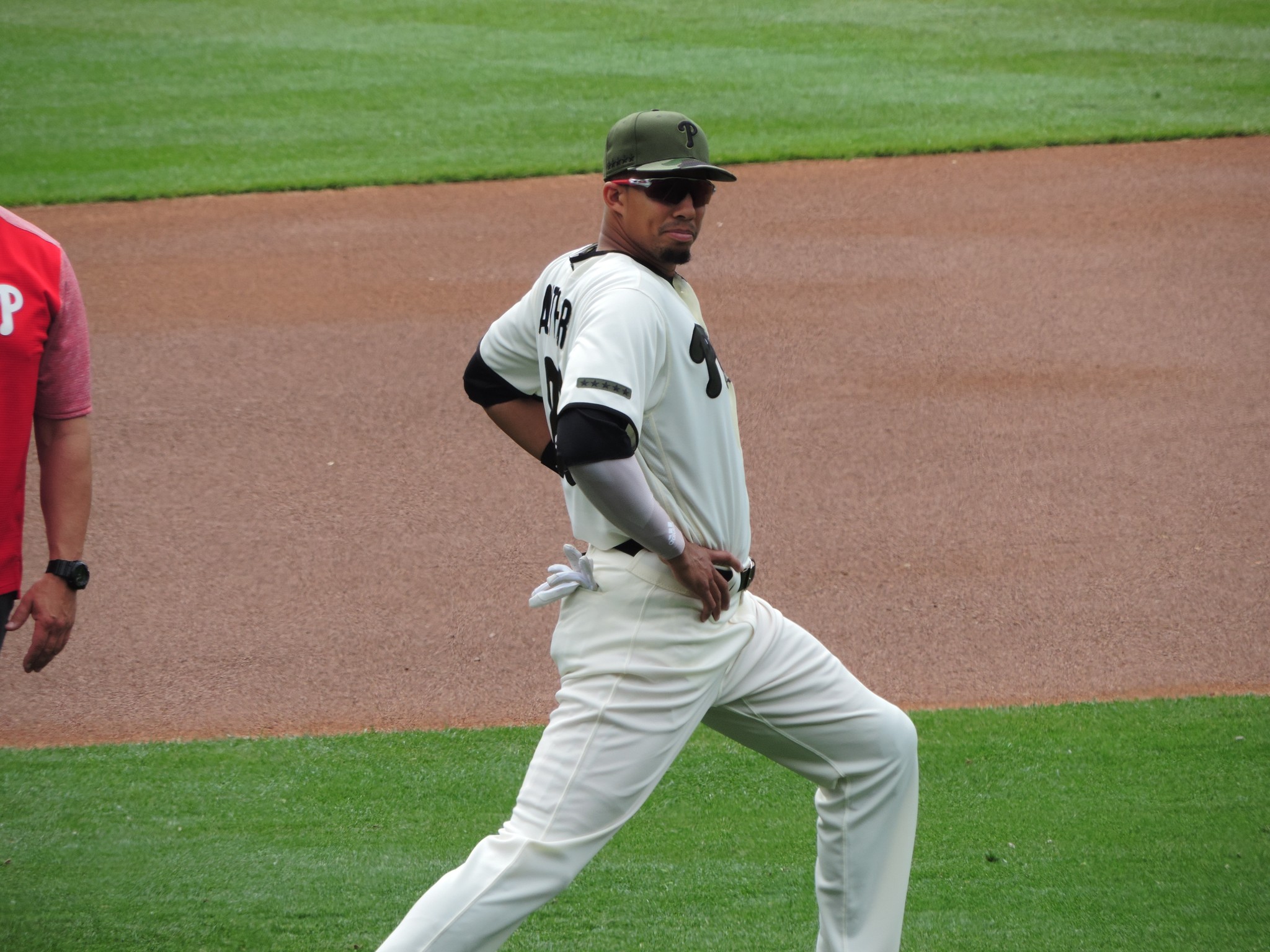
[606,175,718,209]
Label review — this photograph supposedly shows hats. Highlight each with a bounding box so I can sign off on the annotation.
[604,109,739,181]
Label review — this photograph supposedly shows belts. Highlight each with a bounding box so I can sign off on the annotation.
[612,539,756,592]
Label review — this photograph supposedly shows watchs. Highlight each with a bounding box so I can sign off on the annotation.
[44,558,91,591]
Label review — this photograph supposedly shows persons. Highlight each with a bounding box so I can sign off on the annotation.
[371,107,920,951]
[0,204,93,675]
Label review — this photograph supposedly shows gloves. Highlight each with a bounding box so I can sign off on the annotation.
[524,544,601,606]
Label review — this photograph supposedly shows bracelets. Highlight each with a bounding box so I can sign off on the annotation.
[539,440,565,480]
